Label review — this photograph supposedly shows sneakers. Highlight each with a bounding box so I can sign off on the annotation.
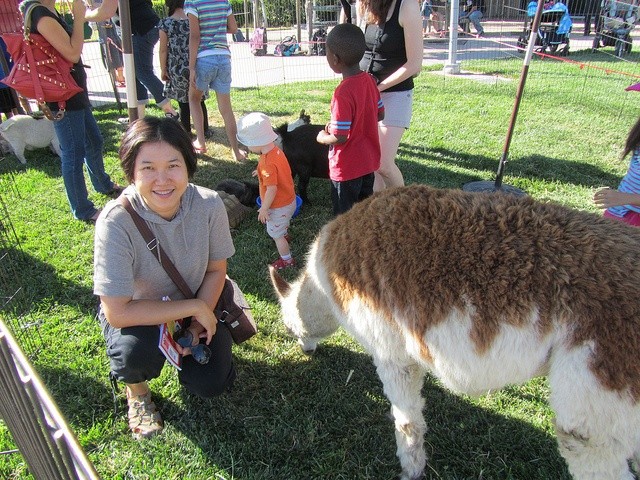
[269,258,298,270]
[85,209,102,224]
[102,184,126,200]
[126,387,163,444]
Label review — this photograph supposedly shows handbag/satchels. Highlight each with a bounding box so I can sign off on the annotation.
[0,4,85,121]
[214,273,257,346]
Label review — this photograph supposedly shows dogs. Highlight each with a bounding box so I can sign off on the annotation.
[272,123,330,202]
[272,109,311,150]
[267,181,640,480]
[215,179,260,207]
[0,114,62,165]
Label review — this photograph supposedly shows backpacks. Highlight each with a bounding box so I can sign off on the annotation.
[309,30,328,55]
[273,34,300,56]
[232,29,245,42]
[248,27,267,56]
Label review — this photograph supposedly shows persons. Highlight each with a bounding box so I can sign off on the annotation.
[20,0,124,224]
[83,1,179,123]
[158,1,213,140]
[94,116,236,438]
[422,1,439,38]
[317,23,386,211]
[355,0,423,191]
[594,115,640,228]
[1,36,30,124]
[456,0,487,39]
[183,0,248,157]
[235,110,298,269]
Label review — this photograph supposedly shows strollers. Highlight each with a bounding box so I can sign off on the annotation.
[517,1,572,53]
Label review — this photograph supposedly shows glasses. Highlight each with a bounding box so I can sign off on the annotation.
[173,328,213,366]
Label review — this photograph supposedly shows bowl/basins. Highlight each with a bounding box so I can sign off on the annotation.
[256,194,303,217]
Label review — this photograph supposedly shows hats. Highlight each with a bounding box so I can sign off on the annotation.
[236,111,278,148]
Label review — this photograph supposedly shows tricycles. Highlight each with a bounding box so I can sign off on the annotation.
[591,1,640,57]
